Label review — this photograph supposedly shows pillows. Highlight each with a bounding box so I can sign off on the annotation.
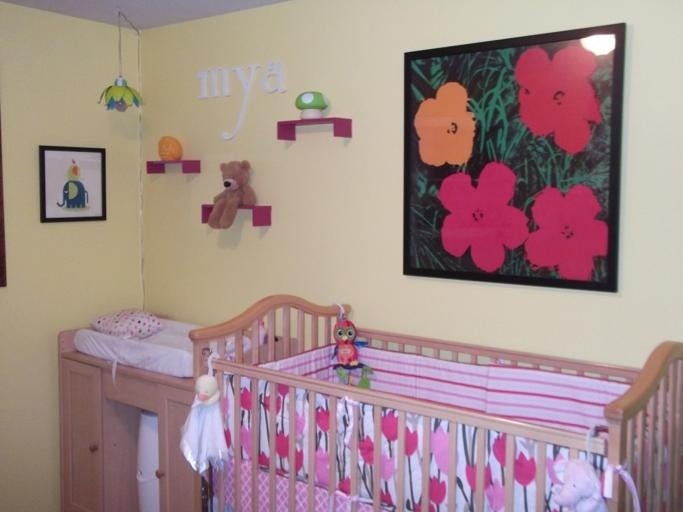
[88,306,164,342]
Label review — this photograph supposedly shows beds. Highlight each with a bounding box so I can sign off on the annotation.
[187,294,681,512]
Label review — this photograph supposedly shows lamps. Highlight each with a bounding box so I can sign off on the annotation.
[96,9,144,113]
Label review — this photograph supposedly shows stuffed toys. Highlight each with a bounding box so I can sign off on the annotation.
[206,159,257,229]
[330,319,369,368]
[178,354,230,482]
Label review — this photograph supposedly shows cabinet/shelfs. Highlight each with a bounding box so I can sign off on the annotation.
[60,353,193,512]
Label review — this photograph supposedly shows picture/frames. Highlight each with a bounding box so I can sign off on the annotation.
[37,145,106,223]
[400,21,627,294]
